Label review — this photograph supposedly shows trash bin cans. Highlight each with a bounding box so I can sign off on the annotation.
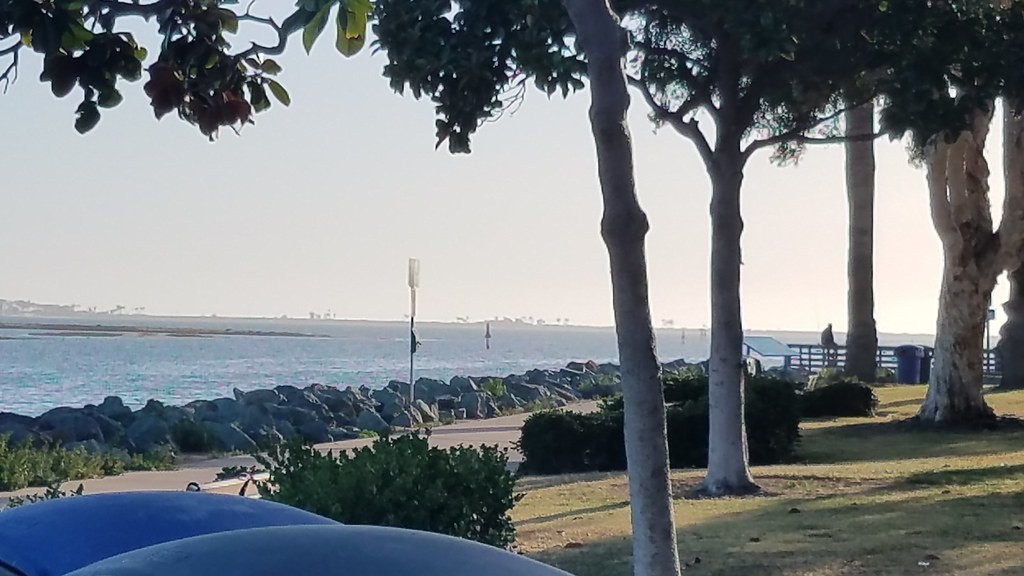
[894,344,924,384]
[917,345,935,384]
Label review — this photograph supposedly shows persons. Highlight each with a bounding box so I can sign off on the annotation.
[821,323,837,359]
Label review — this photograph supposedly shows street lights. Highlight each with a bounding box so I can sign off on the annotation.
[406,256,420,408]
